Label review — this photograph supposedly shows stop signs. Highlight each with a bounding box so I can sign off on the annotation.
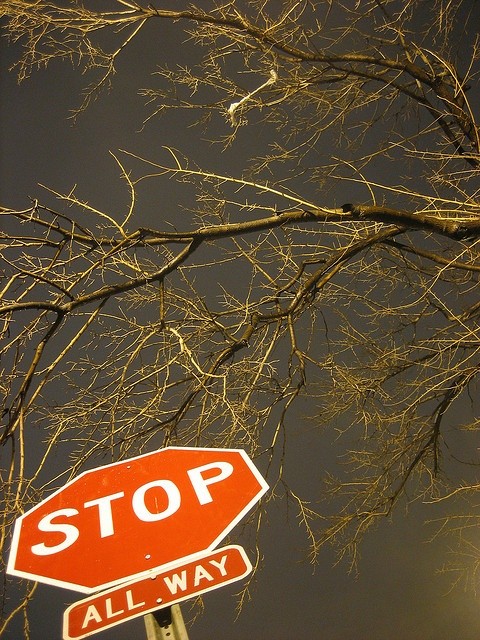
[6,446,270,596]
